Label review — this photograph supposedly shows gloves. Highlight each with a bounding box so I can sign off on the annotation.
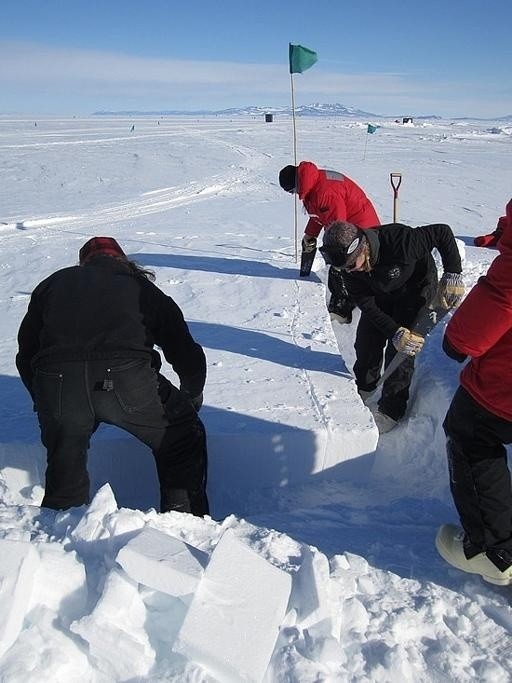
[180,387,204,412]
[391,327,425,356]
[302,235,316,252]
[438,272,465,310]
[443,335,467,362]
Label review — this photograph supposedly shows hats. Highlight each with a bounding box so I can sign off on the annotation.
[279,166,296,192]
[80,237,128,260]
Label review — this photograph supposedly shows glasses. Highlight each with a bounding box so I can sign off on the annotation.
[319,221,364,267]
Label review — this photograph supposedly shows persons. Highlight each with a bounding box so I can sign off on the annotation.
[318,222,466,434]
[434,195,512,587]
[279,160,381,322]
[15,239,209,514]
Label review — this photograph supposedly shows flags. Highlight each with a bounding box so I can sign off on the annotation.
[290,44,318,74]
[368,125,376,133]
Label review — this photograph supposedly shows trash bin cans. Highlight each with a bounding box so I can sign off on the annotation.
[266,114,273,122]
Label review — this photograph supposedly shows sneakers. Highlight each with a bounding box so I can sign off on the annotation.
[330,312,348,324]
[353,388,379,400]
[373,411,398,435]
[435,522,512,586]
